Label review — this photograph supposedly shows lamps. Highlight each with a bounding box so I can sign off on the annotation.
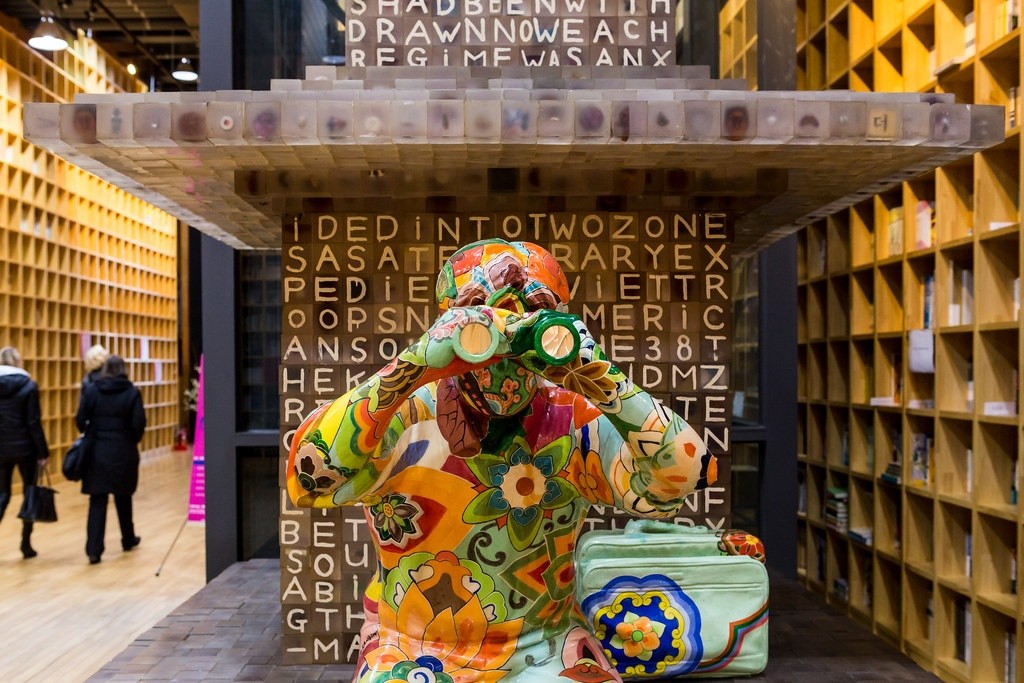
[29,8,69,51]
[172,57,198,82]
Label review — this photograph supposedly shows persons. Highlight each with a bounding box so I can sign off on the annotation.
[75,344,147,565]
[0,348,50,560]
[284,238,718,683]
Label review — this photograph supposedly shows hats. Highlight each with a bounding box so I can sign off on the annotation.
[85,344,111,373]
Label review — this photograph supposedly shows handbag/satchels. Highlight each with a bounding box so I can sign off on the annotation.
[18,468,58,522]
[62,435,85,481]
[574,517,769,679]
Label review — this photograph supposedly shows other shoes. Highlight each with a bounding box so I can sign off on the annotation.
[19,539,37,558]
[87,550,101,565]
[122,536,141,552]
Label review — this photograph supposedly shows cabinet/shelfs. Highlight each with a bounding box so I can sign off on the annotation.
[0,9,180,495]
[717,0,1024,683]
